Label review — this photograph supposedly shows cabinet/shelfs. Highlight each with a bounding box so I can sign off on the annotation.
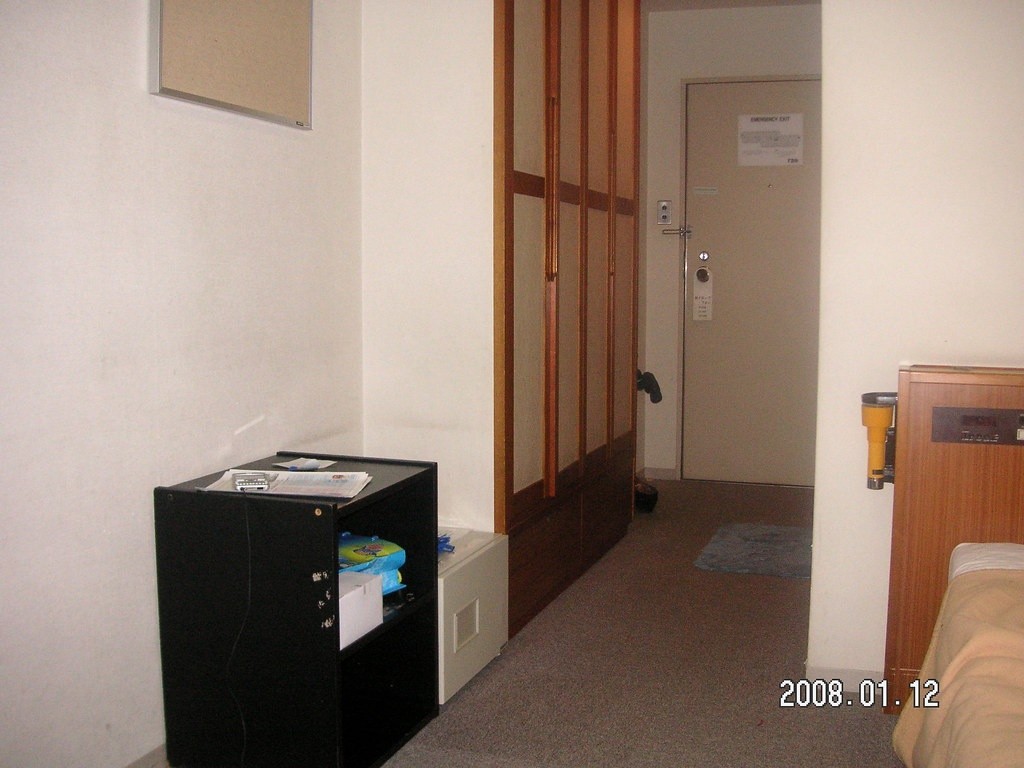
[154,450,440,768]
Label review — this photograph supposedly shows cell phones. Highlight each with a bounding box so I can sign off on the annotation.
[232,472,269,492]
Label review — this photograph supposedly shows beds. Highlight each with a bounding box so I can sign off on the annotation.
[894,542,1024,768]
